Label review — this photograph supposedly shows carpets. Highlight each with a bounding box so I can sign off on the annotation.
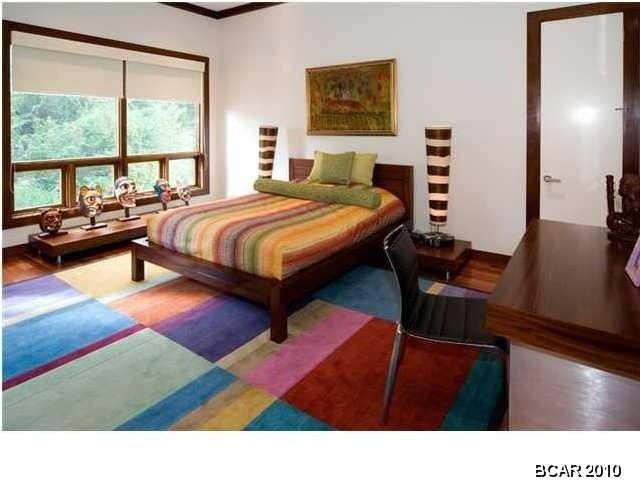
[3,252,509,431]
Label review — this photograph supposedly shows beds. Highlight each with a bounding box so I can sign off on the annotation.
[129,157,415,344]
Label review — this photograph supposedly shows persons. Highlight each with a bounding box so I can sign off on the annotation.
[114,176,137,207]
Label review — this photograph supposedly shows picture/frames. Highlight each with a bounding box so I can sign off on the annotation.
[305,57,398,136]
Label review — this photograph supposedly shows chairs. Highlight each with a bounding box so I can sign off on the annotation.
[381,223,510,421]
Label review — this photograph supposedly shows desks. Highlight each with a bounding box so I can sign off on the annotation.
[28,207,163,264]
[414,237,472,281]
[485,218,639,430]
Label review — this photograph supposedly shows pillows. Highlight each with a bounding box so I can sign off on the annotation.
[306,150,378,187]
[253,178,382,210]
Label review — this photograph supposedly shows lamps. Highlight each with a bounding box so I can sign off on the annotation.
[258,127,278,181]
[419,125,455,247]
